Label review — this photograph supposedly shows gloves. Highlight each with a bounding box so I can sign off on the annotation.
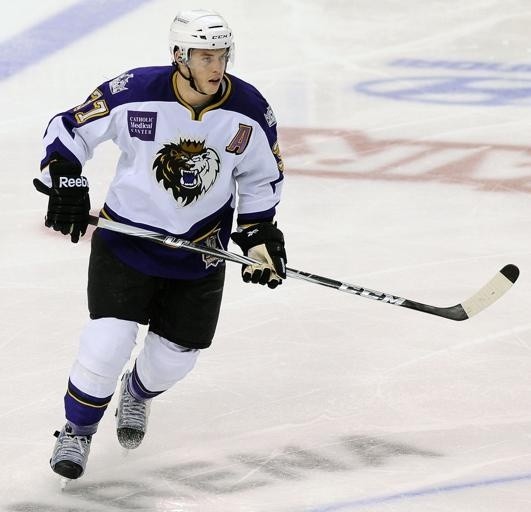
[34,162,88,243]
[231,223,286,289]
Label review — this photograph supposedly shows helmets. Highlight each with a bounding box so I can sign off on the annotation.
[168,8,237,73]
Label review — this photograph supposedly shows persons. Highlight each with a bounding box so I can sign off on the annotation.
[40,11,288,480]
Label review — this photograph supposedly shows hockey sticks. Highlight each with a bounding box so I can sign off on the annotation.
[87,215,520,320]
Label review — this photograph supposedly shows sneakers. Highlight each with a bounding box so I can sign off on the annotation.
[50,421,98,480]
[117,370,149,449]
